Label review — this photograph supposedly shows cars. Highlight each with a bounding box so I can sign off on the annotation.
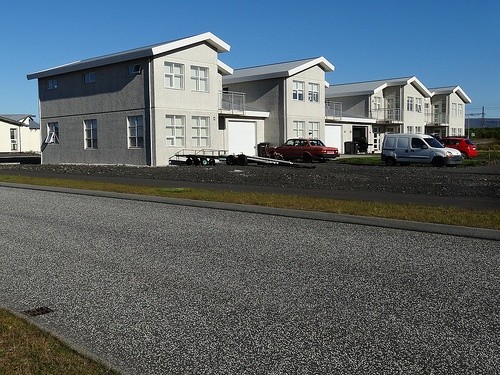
[270,138,338,162]
[440,136,477,158]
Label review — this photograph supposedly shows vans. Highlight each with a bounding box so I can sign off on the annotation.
[382,133,460,168]
